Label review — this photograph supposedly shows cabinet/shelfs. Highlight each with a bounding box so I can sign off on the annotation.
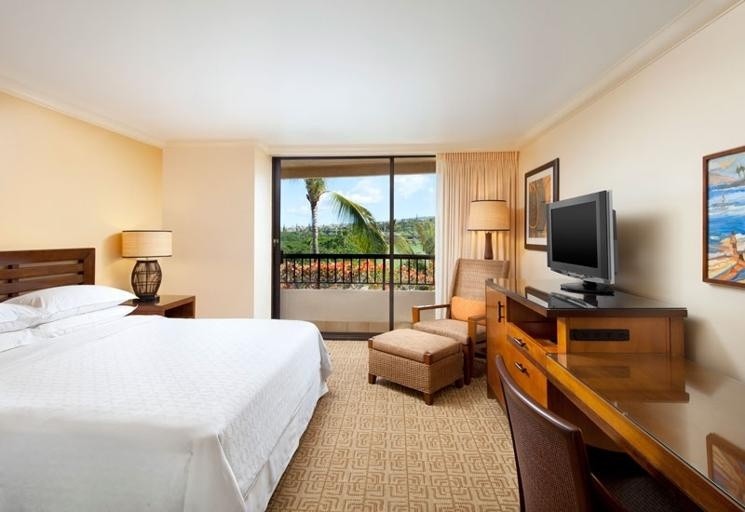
[486,277,687,419]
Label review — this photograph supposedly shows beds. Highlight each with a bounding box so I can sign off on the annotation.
[0,248,333,512]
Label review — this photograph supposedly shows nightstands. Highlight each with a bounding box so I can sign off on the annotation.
[120,295,196,318]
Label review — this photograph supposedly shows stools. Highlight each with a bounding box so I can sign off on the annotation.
[369,328,465,405]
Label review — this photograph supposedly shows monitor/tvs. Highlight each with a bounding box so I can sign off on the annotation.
[546,191,617,295]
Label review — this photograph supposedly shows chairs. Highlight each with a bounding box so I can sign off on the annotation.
[496,353,684,512]
[411,258,510,385]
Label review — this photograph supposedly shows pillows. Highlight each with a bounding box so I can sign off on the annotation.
[33,304,139,342]
[451,296,487,326]
[2,285,140,324]
[0,303,51,333]
[0,328,37,353]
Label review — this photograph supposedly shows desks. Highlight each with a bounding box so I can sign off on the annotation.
[546,354,745,512]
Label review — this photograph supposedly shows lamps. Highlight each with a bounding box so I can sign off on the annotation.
[468,200,511,260]
[121,230,173,304]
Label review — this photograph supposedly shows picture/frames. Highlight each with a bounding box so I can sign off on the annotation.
[524,157,559,252]
[703,146,745,289]
